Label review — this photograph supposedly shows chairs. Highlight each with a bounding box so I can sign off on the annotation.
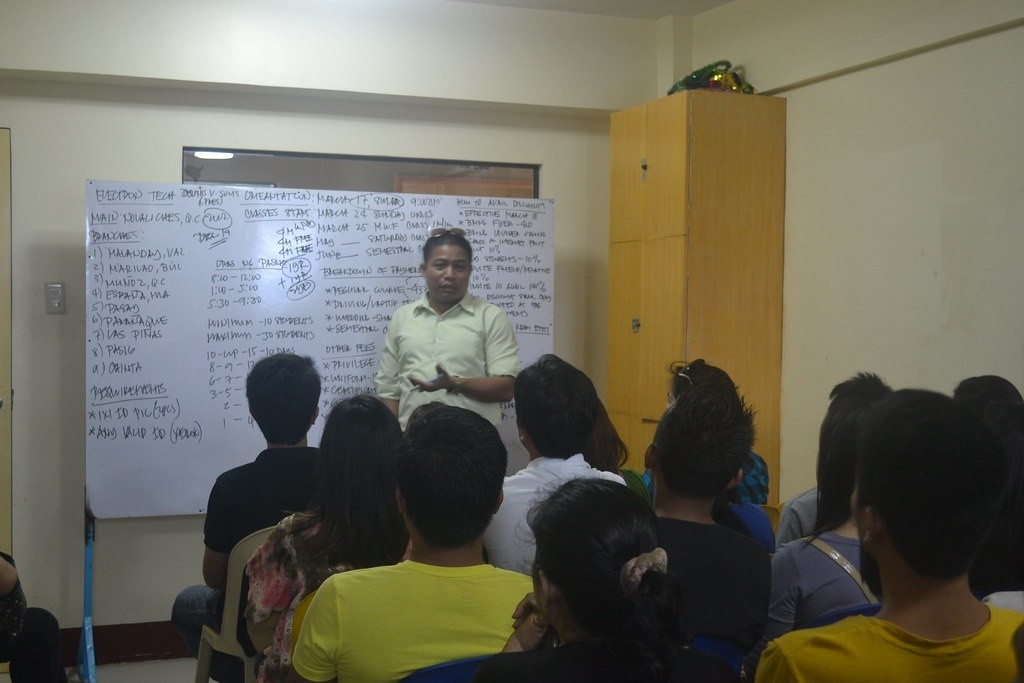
[194,524,272,683]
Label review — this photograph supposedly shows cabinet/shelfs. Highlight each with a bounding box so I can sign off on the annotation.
[604,88,787,508]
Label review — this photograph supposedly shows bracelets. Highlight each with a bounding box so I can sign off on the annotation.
[447,376,461,396]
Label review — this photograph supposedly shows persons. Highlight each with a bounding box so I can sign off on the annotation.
[471,478,740,683]
[774,371,893,548]
[739,392,891,680]
[754,389,1024,683]
[172,354,322,683]
[375,234,520,435]
[646,358,769,505]
[286,402,534,683]
[484,354,629,575]
[952,375,1024,601]
[247,395,411,683]
[0,553,68,683]
[643,381,771,655]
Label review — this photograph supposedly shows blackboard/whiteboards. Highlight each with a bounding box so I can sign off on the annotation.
[82,174,556,520]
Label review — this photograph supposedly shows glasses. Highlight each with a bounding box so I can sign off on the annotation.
[431,227,466,240]
[678,357,705,386]
